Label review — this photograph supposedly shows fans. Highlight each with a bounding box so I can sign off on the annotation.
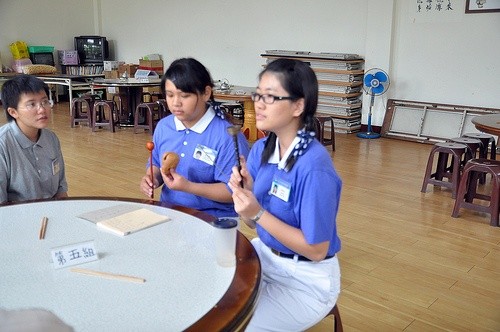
[357,68,390,139]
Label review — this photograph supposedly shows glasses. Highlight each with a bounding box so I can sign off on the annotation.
[250,91,296,104]
[16,99,51,112]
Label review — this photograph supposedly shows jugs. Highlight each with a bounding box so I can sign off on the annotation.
[220,82,231,90]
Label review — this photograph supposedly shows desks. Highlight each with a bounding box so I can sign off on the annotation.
[470,114,500,136]
[0,197,261,332]
[0,72,105,117]
[212,85,266,149]
[89,78,163,126]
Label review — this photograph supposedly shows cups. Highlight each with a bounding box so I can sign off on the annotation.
[211,217,237,270]
[214,88,246,95]
[66,65,103,76]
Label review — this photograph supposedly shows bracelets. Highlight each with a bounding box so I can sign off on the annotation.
[253,208,264,222]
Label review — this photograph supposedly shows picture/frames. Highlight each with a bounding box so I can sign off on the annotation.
[465,0,500,14]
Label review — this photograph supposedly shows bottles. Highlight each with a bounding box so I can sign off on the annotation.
[143,54,160,60]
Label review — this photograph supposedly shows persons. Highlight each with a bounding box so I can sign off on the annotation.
[142,57,252,232]
[0,75,68,205]
[195,151,202,159]
[273,184,277,195]
[228,58,342,332]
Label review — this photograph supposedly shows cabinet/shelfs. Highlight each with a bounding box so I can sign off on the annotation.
[61,64,105,99]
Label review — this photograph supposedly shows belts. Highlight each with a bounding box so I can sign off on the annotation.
[271,247,335,261]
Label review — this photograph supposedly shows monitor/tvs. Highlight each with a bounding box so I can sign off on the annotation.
[74,36,109,65]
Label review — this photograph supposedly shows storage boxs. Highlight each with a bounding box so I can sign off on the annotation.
[57,50,78,65]
[28,46,54,53]
[103,60,164,110]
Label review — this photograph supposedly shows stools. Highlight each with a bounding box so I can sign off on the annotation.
[70,92,171,137]
[421,133,500,228]
[314,115,335,151]
